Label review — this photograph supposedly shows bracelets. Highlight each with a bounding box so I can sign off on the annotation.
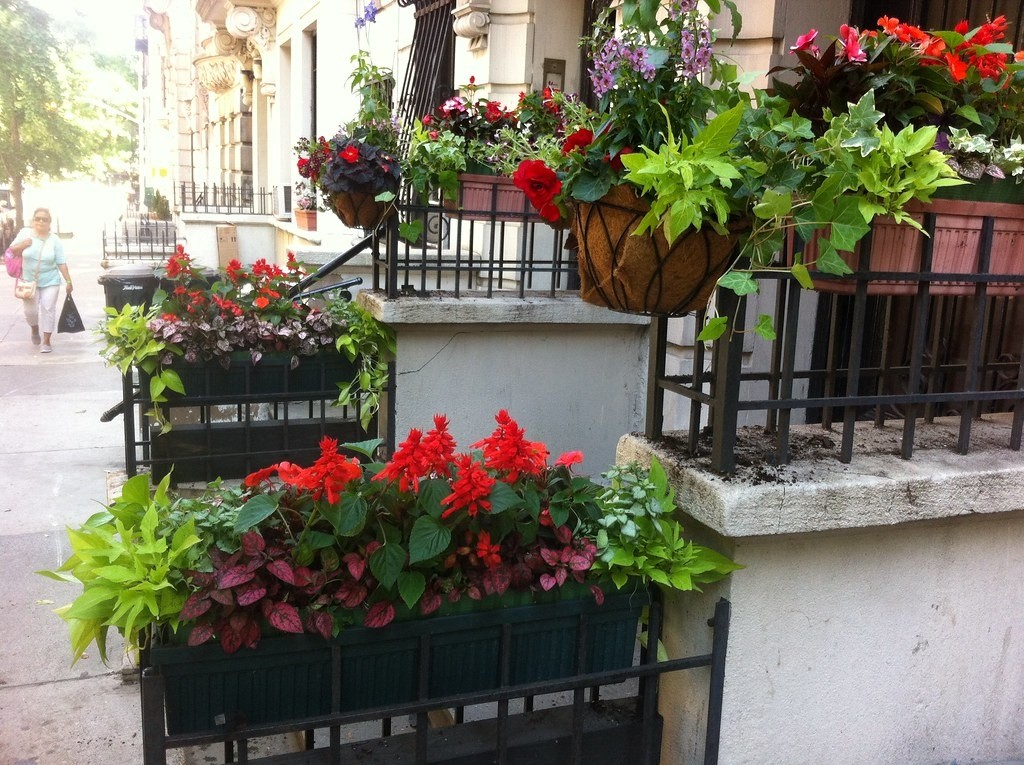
[66,279,72,283]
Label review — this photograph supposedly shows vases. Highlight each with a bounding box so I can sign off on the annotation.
[293,208,317,231]
[443,173,543,223]
[149,592,642,736]
[138,347,366,397]
[804,198,1024,295]
[329,185,399,227]
[562,184,754,317]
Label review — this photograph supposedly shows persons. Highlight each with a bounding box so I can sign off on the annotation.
[8,207,73,353]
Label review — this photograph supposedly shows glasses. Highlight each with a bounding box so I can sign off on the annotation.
[35,216,48,222]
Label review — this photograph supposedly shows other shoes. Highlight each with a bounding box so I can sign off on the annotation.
[41,345,51,352]
[32,334,41,345]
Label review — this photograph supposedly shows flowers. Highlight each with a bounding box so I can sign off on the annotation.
[293,45,404,193]
[38,409,726,668]
[763,14,1024,183]
[506,0,884,338]
[411,76,595,168]
[296,194,317,210]
[93,243,396,437]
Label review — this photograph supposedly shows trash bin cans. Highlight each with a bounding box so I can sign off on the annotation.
[98,265,159,326]
[158,263,222,304]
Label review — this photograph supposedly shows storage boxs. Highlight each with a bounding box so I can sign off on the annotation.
[215,221,238,268]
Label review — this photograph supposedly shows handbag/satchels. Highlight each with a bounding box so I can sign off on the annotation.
[5,248,23,278]
[58,294,85,333]
[15,278,36,299]
